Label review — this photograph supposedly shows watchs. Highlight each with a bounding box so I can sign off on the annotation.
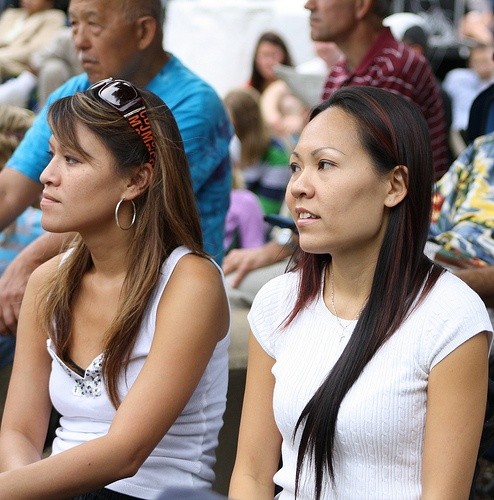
[270,225,296,248]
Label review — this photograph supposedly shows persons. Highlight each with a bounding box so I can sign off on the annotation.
[221,0,449,308]
[229,86,494,499]
[1,1,82,267]
[0,76,233,500]
[219,8,494,425]
[0,0,232,452]
[221,31,313,250]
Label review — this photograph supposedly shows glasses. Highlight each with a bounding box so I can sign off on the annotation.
[87,76,157,168]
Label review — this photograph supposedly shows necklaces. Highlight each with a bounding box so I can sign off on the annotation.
[329,265,371,342]
